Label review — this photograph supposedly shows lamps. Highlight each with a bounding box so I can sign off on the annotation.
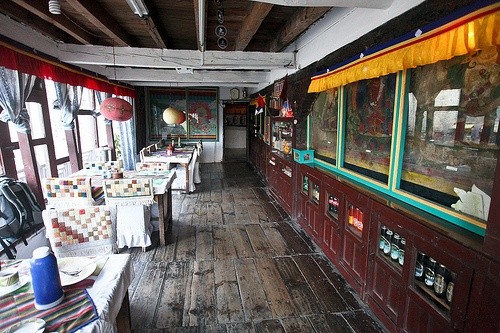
[100,39,133,122]
[126,0,149,19]
[242,88,248,97]
[161,82,186,126]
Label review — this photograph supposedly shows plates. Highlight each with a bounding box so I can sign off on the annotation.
[58,256,97,287]
[0,275,29,297]
[1,317,46,333]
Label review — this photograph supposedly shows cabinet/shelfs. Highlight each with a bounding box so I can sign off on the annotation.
[244,75,500,333]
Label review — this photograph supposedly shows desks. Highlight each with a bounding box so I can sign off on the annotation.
[0,251,136,333]
[71,167,177,248]
[144,142,196,194]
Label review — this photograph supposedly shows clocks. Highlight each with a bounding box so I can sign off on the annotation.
[229,88,239,100]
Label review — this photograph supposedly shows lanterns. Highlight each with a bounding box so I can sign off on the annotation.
[100,97,133,121]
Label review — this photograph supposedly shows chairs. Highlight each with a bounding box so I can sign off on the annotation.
[135,161,170,170]
[139,146,147,162]
[106,178,154,252]
[147,143,155,154]
[40,205,119,256]
[155,139,163,151]
[172,148,198,192]
[84,161,124,170]
[194,140,203,185]
[40,175,104,206]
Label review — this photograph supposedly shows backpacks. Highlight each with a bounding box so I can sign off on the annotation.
[0,174,45,238]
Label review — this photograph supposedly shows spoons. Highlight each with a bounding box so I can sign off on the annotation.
[60,254,105,276]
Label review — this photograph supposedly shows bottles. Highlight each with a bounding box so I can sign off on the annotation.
[348,204,364,232]
[29,246,64,310]
[313,185,319,200]
[378,225,406,267]
[167,144,173,156]
[328,193,339,213]
[415,251,457,305]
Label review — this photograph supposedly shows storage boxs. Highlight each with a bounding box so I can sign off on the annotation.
[293,147,315,164]
